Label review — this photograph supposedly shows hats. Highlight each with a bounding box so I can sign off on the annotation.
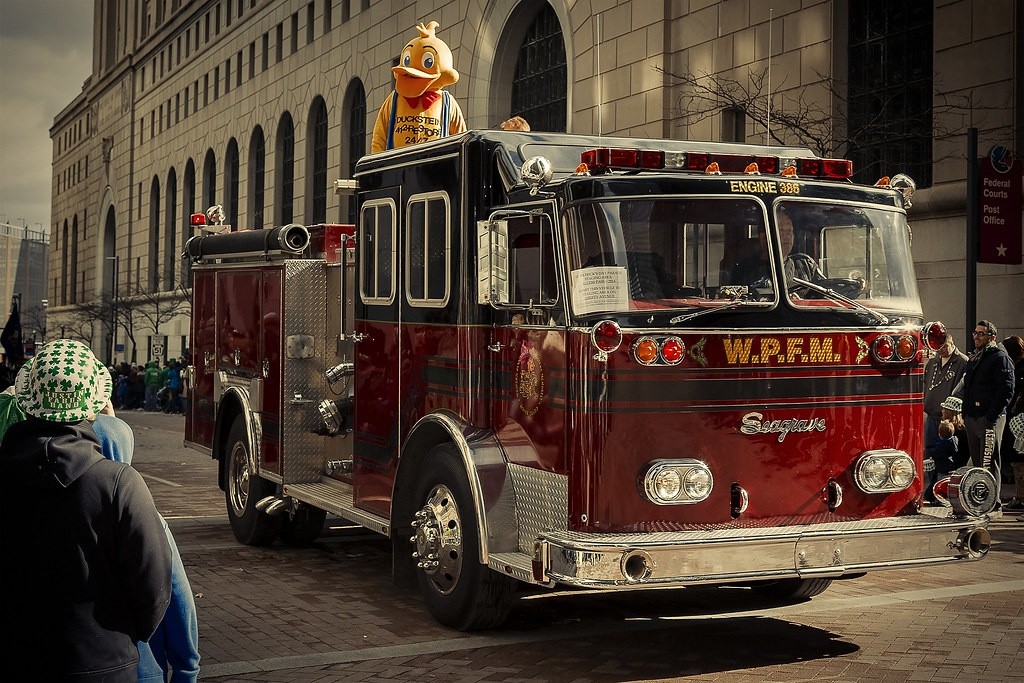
[940,396,962,412]
[15,339,114,423]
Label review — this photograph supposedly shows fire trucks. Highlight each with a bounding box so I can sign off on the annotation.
[181,128,998,635]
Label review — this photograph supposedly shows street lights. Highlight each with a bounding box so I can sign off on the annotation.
[106,256,119,366]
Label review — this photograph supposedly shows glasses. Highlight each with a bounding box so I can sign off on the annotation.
[972,330,992,336]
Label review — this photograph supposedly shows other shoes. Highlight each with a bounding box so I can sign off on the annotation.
[1015,515,1024,522]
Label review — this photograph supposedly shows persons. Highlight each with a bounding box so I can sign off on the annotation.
[938,396,967,474]
[0,339,201,683]
[930,419,960,501]
[921,333,970,501]
[999,335,1024,521]
[580,197,691,302]
[954,320,1015,520]
[734,207,871,301]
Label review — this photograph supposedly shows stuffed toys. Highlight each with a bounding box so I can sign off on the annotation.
[370,20,467,155]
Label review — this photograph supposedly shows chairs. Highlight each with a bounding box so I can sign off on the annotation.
[581,251,674,301]
[719,238,762,286]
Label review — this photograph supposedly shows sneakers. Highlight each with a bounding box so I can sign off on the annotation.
[1003,498,1024,510]
